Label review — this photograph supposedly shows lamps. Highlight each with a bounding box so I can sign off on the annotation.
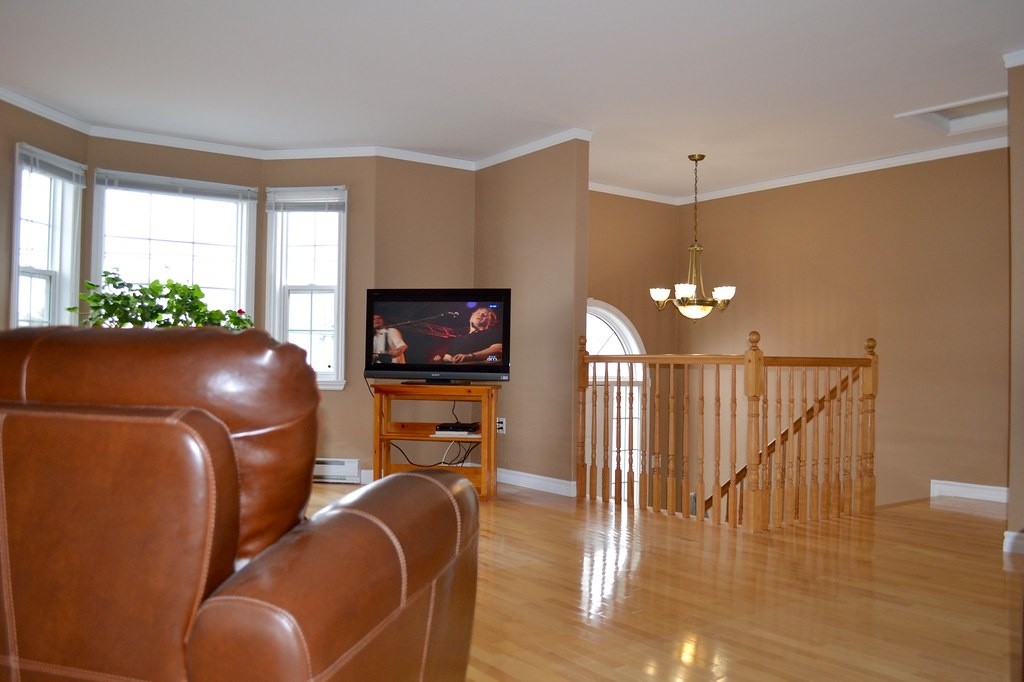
[648,154,736,324]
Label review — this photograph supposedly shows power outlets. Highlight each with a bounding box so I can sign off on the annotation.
[500,418,506,434]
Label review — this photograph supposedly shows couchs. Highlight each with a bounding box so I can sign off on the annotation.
[0,324,480,682]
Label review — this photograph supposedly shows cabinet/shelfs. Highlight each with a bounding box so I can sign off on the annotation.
[369,382,501,502]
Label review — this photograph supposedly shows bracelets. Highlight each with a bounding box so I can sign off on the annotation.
[470,352,476,360]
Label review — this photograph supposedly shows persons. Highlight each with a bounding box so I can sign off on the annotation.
[442,307,502,364]
[371,314,408,364]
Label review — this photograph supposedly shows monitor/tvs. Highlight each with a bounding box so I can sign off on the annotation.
[365,288,511,385]
